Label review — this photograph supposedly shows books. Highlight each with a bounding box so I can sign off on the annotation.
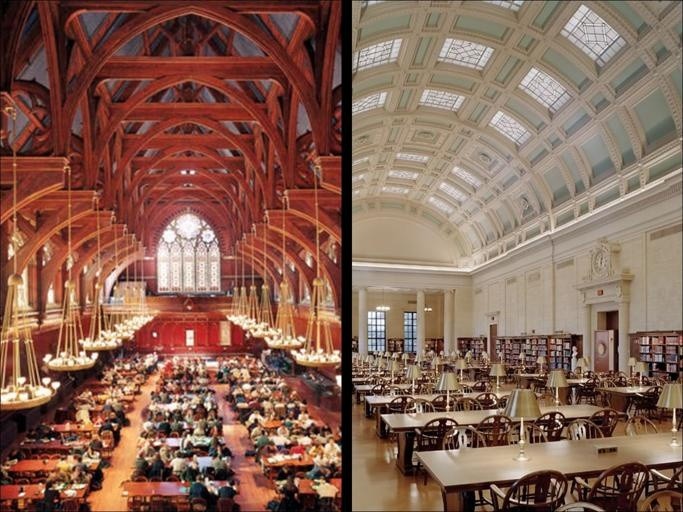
[458,339,571,373]
[638,335,683,380]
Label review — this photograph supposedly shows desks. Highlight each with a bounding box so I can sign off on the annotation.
[0,359,342,499]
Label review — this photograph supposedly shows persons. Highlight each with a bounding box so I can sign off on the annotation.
[0,354,342,512]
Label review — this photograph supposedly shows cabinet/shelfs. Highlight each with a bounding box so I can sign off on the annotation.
[496,334,583,374]
[628,330,682,381]
[457,337,487,360]
[425,337,444,354]
[388,338,403,352]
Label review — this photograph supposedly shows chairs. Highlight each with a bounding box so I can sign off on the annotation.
[126,495,233,511]
[352,350,683,512]
[299,491,333,511]
[61,498,80,511]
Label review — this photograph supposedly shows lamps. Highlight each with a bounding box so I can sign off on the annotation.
[225,168,342,368]
[0,99,154,411]
